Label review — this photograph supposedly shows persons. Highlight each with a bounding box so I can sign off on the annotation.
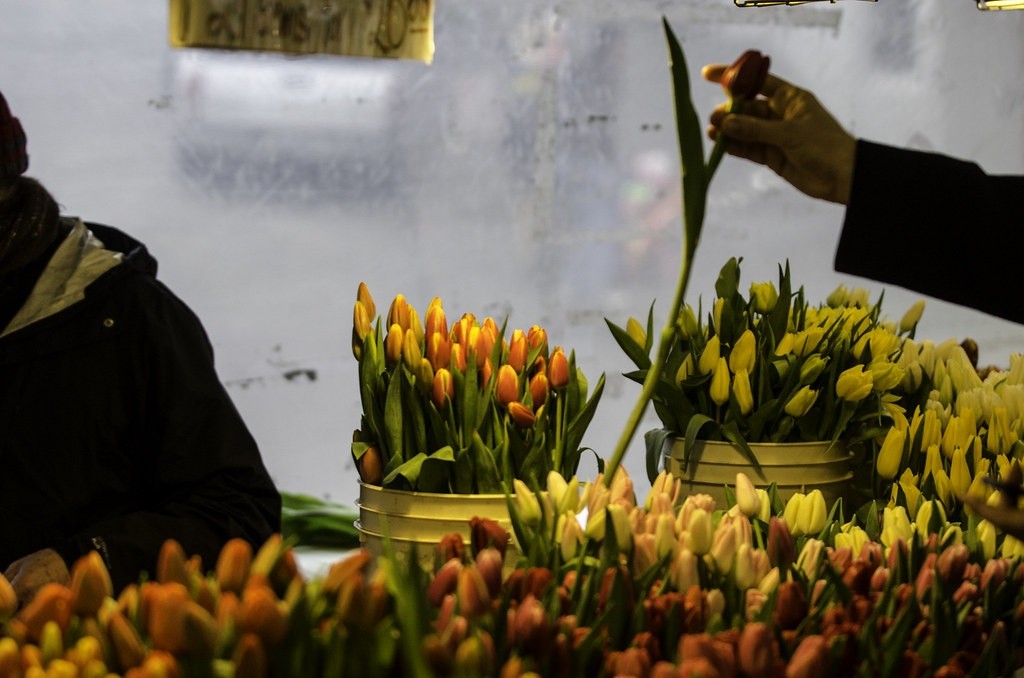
[1,94,281,608]
[700,66,1024,326]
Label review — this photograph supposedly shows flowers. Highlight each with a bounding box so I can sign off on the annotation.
[0,16,1024,678]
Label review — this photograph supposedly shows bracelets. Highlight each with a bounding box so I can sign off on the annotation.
[91,538,110,570]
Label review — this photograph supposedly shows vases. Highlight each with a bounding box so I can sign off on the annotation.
[662,436,853,520]
[352,479,550,561]
